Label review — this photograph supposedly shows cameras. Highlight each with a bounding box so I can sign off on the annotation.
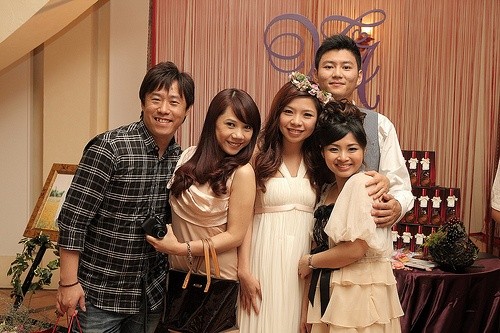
[141,215,168,239]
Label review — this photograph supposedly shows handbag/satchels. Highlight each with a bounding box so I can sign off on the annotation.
[157,238,241,333]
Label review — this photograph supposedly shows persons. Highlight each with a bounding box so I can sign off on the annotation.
[54,60,195,332]
[488,156,500,225]
[234,69,391,333]
[298,99,405,333]
[309,34,414,227]
[148,88,262,332]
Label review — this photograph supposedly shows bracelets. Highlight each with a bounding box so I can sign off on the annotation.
[186,241,193,263]
[57,280,80,288]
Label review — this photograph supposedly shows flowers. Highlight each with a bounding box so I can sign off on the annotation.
[288,71,332,106]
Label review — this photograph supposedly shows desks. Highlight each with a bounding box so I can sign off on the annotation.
[393,253,500,333]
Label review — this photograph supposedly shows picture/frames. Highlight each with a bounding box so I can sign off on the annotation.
[23,162,81,244]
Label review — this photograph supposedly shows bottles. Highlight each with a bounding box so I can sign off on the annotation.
[405,196,416,224]
[414,226,426,252]
[445,188,458,221]
[392,225,399,251]
[431,189,442,224]
[429,227,437,235]
[402,226,412,250]
[417,189,430,224]
[409,151,418,185]
[420,152,430,187]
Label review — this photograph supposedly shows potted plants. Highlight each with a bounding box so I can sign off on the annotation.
[424,218,480,273]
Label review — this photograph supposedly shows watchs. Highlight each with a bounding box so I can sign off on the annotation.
[307,253,316,270]
